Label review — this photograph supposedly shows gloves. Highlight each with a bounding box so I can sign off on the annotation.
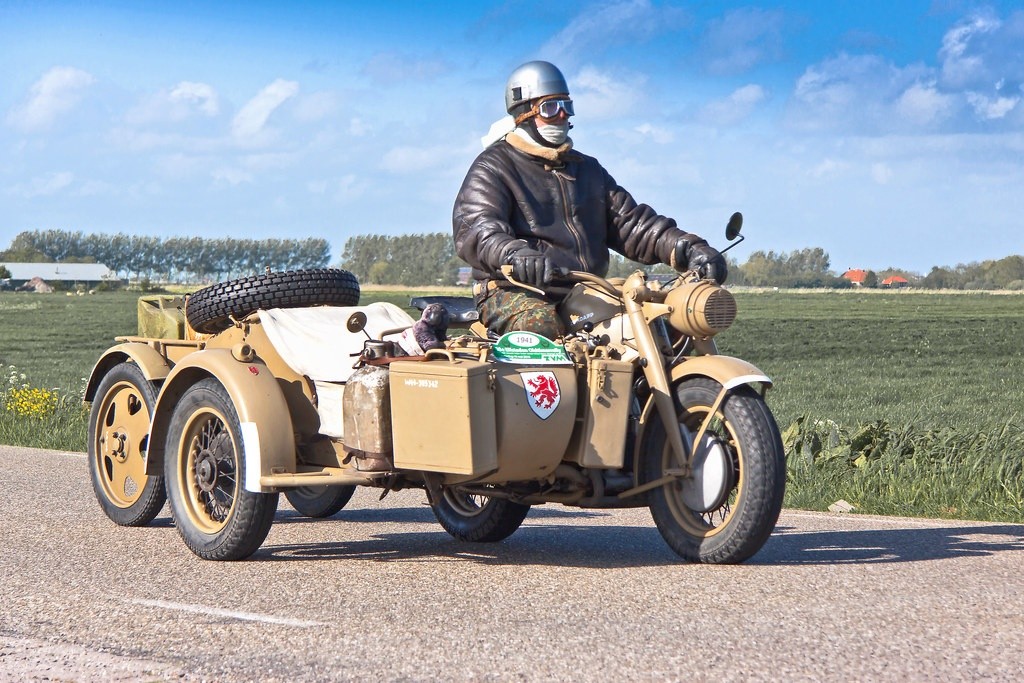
[499,239,569,287]
[674,234,728,286]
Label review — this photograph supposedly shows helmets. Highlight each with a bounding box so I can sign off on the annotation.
[506,61,570,115]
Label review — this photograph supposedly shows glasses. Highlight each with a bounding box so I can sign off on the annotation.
[515,100,575,125]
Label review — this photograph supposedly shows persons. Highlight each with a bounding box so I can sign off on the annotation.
[453,62,728,344]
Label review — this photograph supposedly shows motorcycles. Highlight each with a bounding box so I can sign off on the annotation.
[83,211,787,565]
